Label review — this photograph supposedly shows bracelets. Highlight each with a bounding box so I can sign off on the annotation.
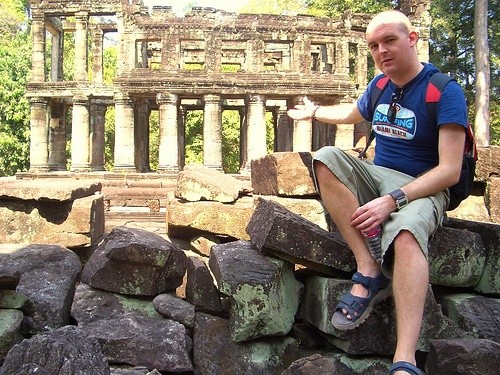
[312,106,321,121]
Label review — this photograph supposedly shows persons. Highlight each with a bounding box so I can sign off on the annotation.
[282,10,473,375]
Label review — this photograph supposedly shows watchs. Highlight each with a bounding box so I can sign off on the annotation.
[388,188,408,212]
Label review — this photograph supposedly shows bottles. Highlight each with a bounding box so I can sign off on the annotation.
[360,224,382,262]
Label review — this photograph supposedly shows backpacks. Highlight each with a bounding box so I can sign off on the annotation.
[368,72,480,212]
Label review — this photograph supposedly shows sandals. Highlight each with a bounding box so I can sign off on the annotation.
[387,355,425,375]
[329,263,392,331]
